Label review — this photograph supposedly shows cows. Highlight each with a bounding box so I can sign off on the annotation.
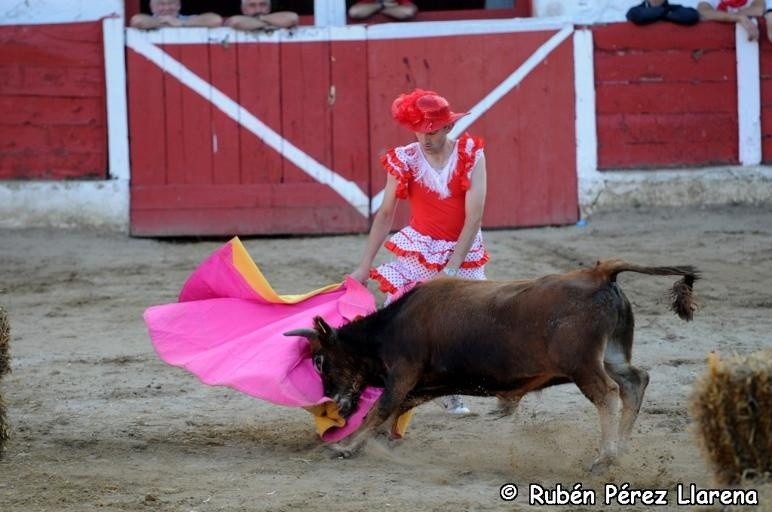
[281,259,702,475]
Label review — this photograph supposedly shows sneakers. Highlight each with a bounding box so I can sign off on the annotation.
[433,394,470,414]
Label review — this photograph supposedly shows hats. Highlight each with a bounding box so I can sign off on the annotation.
[392,88,470,133]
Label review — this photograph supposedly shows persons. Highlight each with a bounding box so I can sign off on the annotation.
[346,1,421,25]
[125,0,223,28]
[624,0,772,42]
[340,87,488,420]
[221,0,301,32]
